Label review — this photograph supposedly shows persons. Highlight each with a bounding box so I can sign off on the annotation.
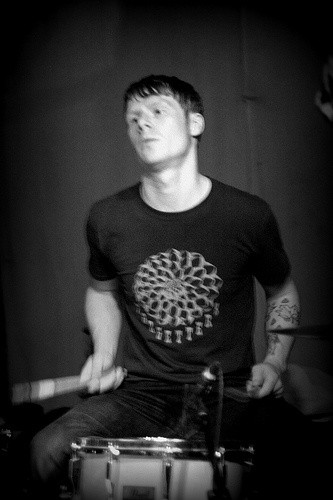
[23,72,333,500]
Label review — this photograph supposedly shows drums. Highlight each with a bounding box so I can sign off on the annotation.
[65,432,254,500]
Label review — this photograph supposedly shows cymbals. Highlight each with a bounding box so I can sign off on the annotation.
[268,327,333,338]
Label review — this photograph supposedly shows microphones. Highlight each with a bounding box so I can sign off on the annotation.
[159,370,214,411]
